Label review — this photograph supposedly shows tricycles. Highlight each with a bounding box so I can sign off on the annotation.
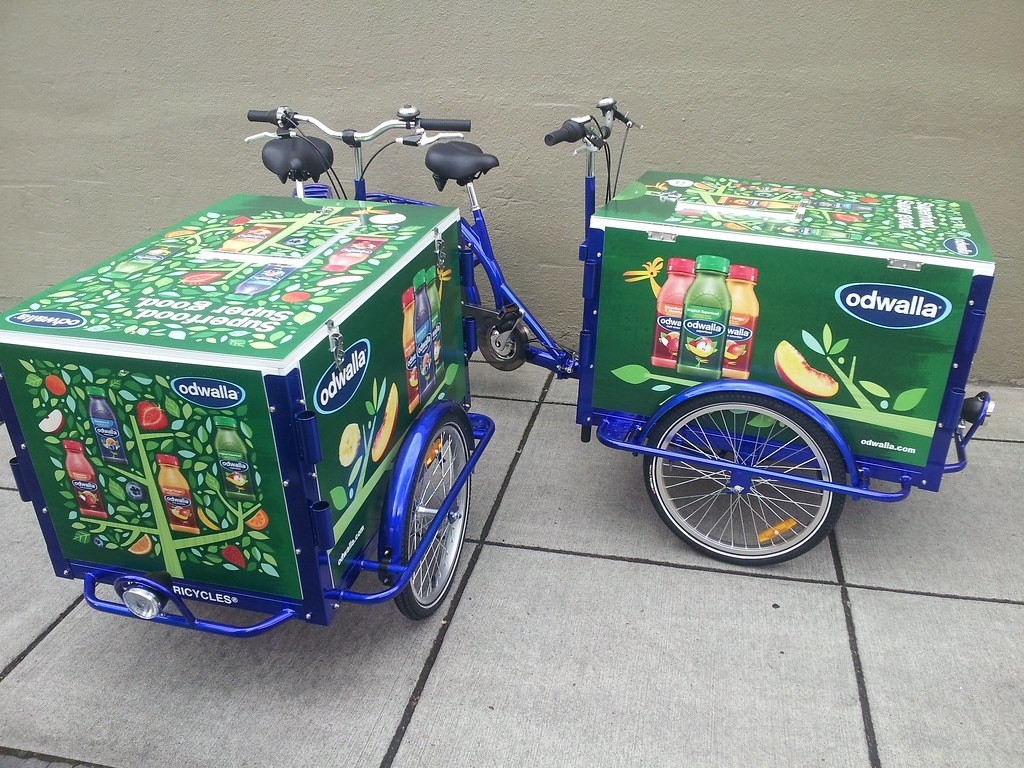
[0,106,497,640]
[423,96,997,567]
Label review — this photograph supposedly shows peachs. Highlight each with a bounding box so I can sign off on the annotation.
[773,339,839,397]
[372,383,398,462]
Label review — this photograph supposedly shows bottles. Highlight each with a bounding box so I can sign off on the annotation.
[214,415,257,502]
[224,263,298,296]
[717,196,799,210]
[809,198,875,212]
[732,182,814,198]
[402,285,419,414]
[651,257,696,370]
[217,224,287,252]
[425,265,446,385]
[320,237,388,272]
[677,255,732,380]
[61,439,108,518]
[86,386,128,464]
[721,265,759,380]
[413,268,437,404]
[157,454,200,534]
[763,222,863,242]
[106,242,189,279]
